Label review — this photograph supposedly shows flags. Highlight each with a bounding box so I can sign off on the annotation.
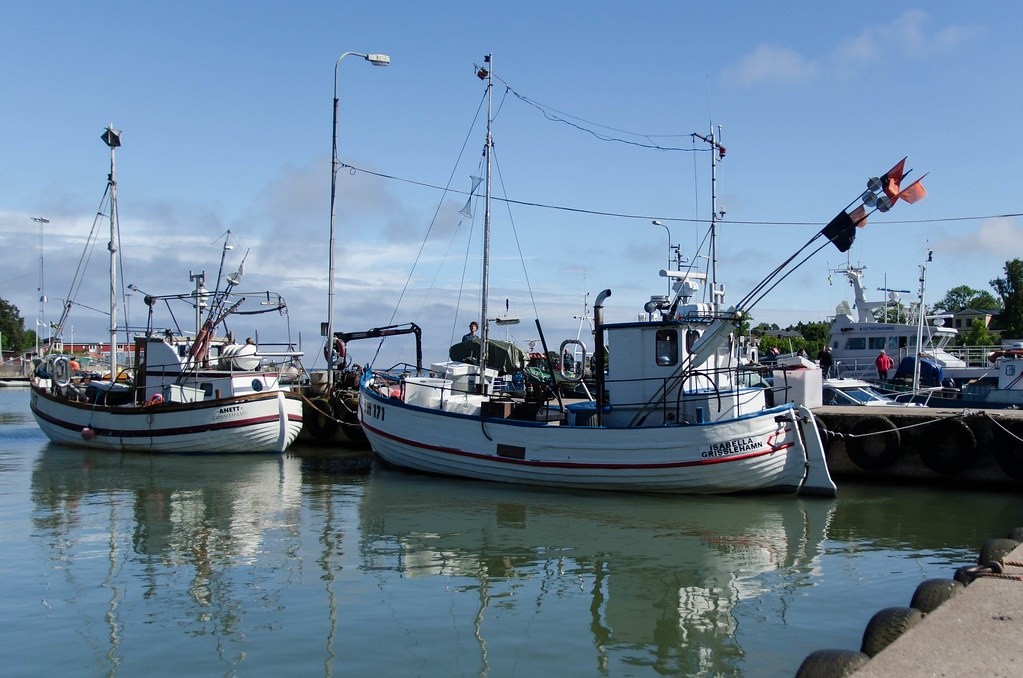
[896,180,927,206]
[821,210,857,253]
[849,207,867,228]
[880,160,905,207]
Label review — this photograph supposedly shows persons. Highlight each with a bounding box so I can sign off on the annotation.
[820,347,833,379]
[462,322,480,342]
[590,351,596,377]
[765,345,787,358]
[818,345,827,367]
[245,337,261,371]
[678,313,682,321]
[797,349,805,358]
[875,349,890,381]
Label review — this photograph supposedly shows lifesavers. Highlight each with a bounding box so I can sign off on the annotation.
[323,336,347,364]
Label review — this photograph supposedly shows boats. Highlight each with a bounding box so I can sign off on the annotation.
[354,53,811,497]
[747,239,1023,408]
[27,127,304,454]
[355,462,792,678]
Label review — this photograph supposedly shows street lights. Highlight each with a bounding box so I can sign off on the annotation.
[326,46,392,415]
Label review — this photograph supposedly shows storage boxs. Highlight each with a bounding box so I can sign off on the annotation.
[772,369,823,409]
[163,384,206,403]
[404,377,454,410]
